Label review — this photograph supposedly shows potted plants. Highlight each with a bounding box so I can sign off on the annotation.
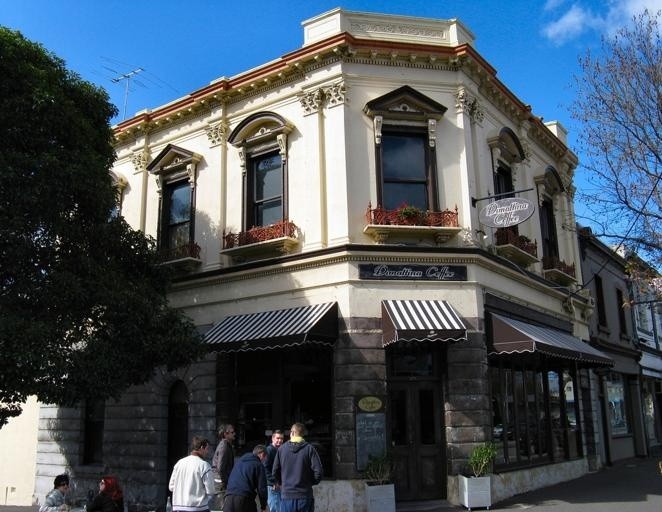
[457,441,499,512]
[361,451,403,512]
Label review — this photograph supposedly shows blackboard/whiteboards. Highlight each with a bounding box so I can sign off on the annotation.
[354,410,390,473]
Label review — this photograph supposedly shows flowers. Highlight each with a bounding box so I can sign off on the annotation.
[381,200,432,226]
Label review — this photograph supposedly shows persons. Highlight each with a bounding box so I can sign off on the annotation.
[271,421,324,512]
[85,475,124,512]
[210,421,236,511]
[260,426,282,511]
[220,443,268,512]
[39,474,72,512]
[168,435,219,512]
